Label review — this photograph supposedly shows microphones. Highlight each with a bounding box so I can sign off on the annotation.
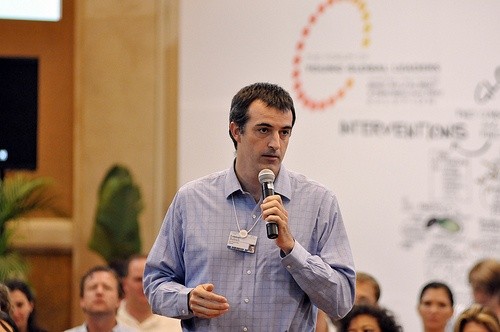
[259,168,279,239]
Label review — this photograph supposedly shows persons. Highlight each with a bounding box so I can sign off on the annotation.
[315,272,403,332]
[453,259,500,332]
[143,83,356,332]
[416,282,455,332]
[0,277,47,332]
[116,255,182,332]
[64,266,137,332]
